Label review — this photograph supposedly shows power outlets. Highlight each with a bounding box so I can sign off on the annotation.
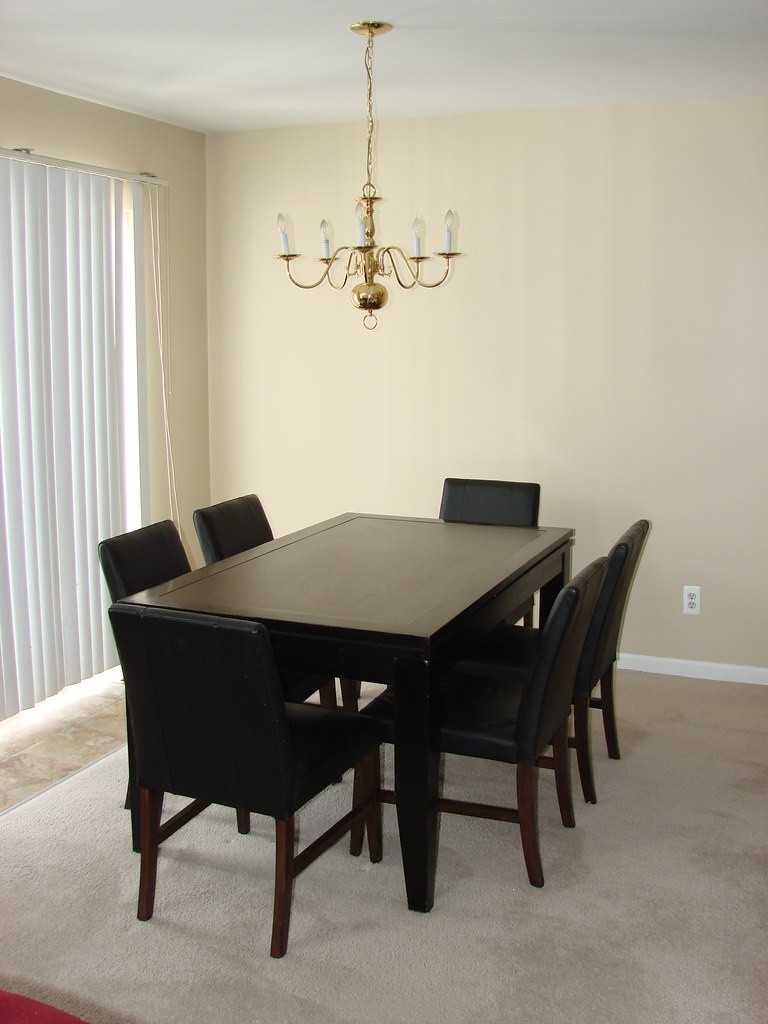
[683,586,700,614]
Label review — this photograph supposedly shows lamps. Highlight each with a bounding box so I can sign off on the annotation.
[272,21,462,331]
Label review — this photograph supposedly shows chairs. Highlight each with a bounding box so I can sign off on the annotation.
[96,478,651,960]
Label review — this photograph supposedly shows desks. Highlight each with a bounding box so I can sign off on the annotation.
[116,512,575,913]
